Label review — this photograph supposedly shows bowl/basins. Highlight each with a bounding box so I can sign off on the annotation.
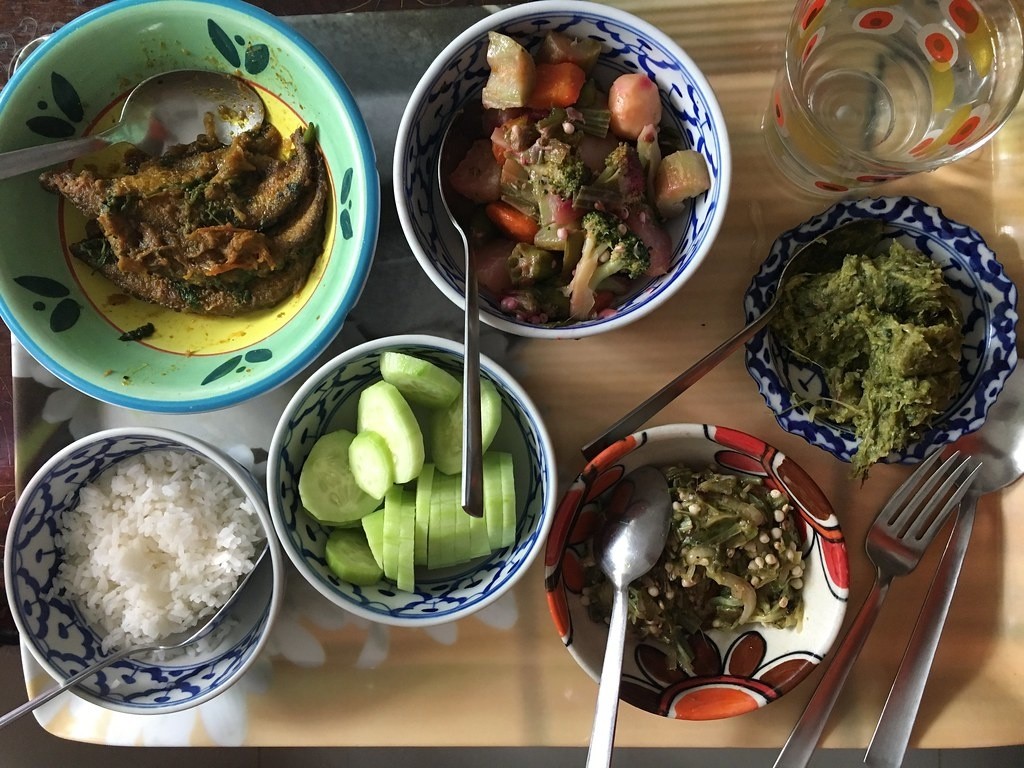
[744,196,1019,464]
[4,427,285,714]
[0,1,382,413]
[391,0,734,342]
[268,336,557,625]
[543,424,851,722]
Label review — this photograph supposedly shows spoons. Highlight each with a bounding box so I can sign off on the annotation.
[0,70,267,180]
[0,537,268,732]
[863,357,1024,768]
[437,91,503,519]
[581,464,672,768]
[580,212,892,464]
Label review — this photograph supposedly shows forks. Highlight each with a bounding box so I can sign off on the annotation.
[770,444,985,768]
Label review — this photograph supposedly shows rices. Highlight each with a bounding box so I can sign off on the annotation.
[49,451,267,687]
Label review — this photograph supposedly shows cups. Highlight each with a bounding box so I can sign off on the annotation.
[759,0,1024,196]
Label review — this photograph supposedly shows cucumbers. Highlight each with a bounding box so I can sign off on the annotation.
[295,349,518,592]
